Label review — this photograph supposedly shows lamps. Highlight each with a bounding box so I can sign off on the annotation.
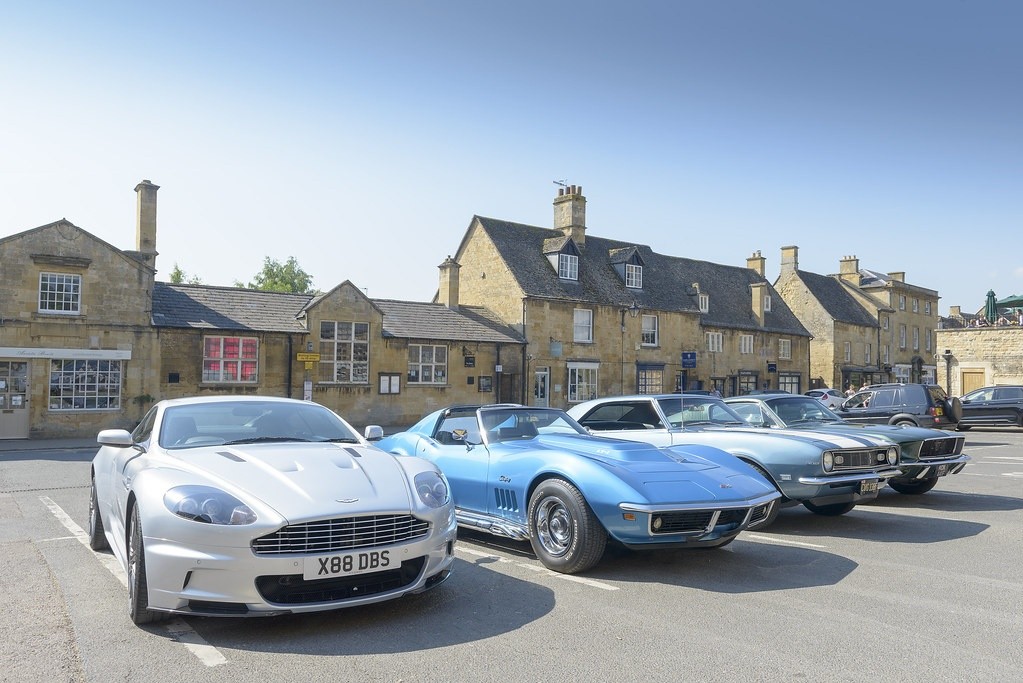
[630,303,639,317]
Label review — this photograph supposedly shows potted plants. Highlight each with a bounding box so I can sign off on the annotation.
[132,393,155,425]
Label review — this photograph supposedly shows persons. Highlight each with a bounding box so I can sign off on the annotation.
[964,309,1023,328]
[845,383,869,396]
[709,386,724,398]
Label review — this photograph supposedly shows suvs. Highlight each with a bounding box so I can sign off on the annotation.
[956,386,1023,431]
[803,382,963,432]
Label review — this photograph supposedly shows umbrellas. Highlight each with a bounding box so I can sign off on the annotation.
[983,289,1023,325]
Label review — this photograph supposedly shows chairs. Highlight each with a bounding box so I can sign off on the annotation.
[165,417,196,447]
[255,415,294,438]
[436,431,473,445]
[497,427,523,441]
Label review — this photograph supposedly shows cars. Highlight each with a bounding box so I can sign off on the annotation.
[804,388,849,410]
[749,389,791,394]
[373,402,783,576]
[658,393,972,506]
[90,395,457,627]
[523,393,903,530]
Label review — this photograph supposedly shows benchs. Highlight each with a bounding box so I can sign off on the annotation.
[581,420,647,430]
